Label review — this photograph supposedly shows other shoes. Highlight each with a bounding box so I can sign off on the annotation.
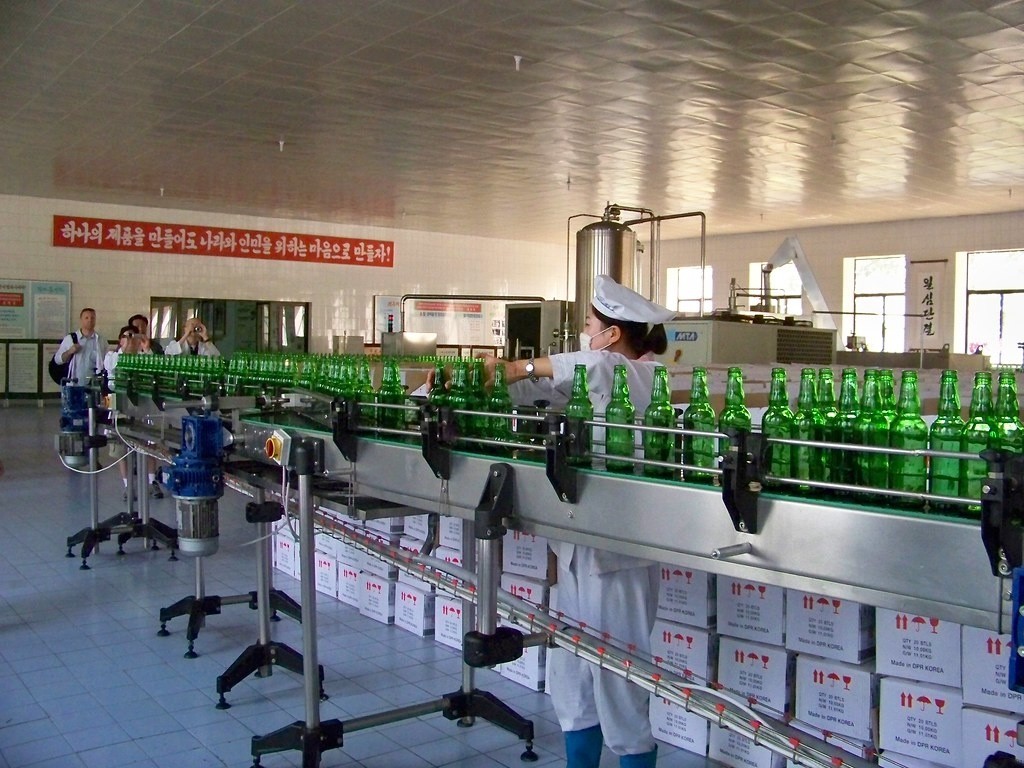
[122,486,136,501]
[134,464,138,474]
[149,480,163,499]
[96,463,104,469]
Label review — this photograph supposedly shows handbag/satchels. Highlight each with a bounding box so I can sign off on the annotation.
[48,332,79,385]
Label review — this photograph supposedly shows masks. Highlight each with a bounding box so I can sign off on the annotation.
[579,325,618,353]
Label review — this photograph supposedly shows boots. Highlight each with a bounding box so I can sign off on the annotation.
[619,743,658,768]
[563,723,603,768]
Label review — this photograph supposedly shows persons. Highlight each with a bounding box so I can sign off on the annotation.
[425,274,683,768]
[161,317,221,356]
[126,314,165,476]
[53,306,110,456]
[101,324,166,501]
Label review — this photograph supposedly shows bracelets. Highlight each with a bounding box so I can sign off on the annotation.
[204,338,210,343]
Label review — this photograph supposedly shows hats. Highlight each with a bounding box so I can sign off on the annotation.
[591,273,679,325]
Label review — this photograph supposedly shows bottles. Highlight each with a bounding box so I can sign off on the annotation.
[718,366,752,484]
[642,366,677,475]
[993,371,1024,493]
[927,368,968,512]
[116,353,513,447]
[795,359,929,507]
[757,368,795,488]
[959,372,1001,515]
[564,363,594,465]
[605,364,635,469]
[683,368,715,482]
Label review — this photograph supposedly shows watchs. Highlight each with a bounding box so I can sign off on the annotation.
[525,357,539,383]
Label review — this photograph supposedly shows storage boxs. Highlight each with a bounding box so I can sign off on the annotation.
[273,364,1024,767]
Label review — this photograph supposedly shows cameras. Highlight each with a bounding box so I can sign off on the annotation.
[127,333,142,339]
[194,326,202,333]
